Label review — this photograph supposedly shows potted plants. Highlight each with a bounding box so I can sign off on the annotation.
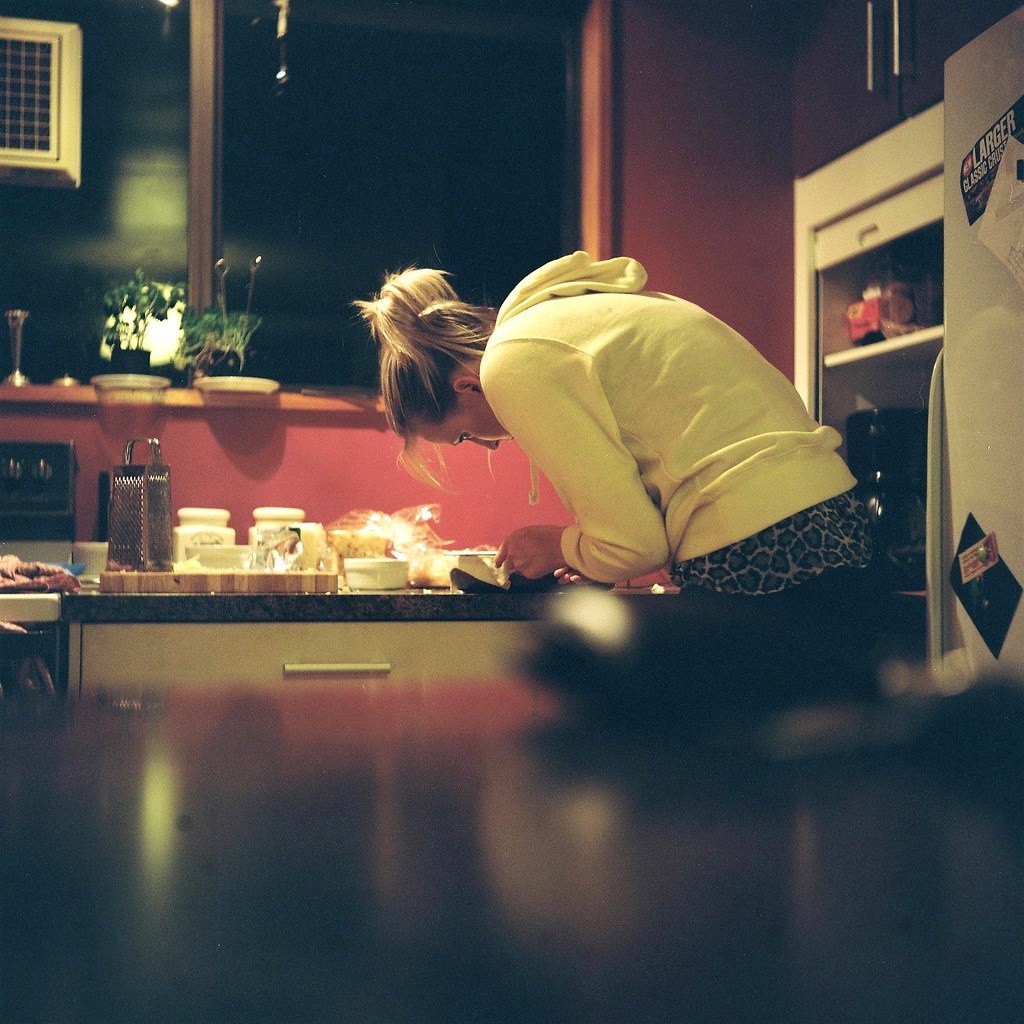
[100,264,264,375]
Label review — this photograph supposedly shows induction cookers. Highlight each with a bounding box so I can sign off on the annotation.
[0,440,77,623]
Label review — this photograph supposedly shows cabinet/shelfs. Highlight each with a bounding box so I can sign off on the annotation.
[796,100,944,429]
[62,590,682,688]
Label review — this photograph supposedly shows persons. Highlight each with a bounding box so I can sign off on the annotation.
[352,250,884,596]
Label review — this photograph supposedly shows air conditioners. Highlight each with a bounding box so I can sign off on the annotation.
[0,16,84,190]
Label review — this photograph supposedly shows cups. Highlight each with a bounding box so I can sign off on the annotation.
[73,542,109,577]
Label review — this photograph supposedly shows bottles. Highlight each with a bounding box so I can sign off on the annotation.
[172,508,235,564]
[248,507,305,545]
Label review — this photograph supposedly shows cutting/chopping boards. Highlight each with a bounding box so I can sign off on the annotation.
[98,572,338,593]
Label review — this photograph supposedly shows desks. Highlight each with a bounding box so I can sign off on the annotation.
[0,683,1024,1024]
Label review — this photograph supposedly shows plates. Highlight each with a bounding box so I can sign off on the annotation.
[194,376,280,395]
[88,375,171,388]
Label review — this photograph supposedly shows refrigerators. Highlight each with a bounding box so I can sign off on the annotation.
[943,7,1024,687]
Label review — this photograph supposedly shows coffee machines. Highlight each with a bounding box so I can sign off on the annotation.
[845,408,924,595]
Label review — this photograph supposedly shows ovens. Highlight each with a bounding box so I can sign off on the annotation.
[0,623,68,699]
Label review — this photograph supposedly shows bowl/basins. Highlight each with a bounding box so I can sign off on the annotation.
[188,545,252,568]
[344,557,409,589]
